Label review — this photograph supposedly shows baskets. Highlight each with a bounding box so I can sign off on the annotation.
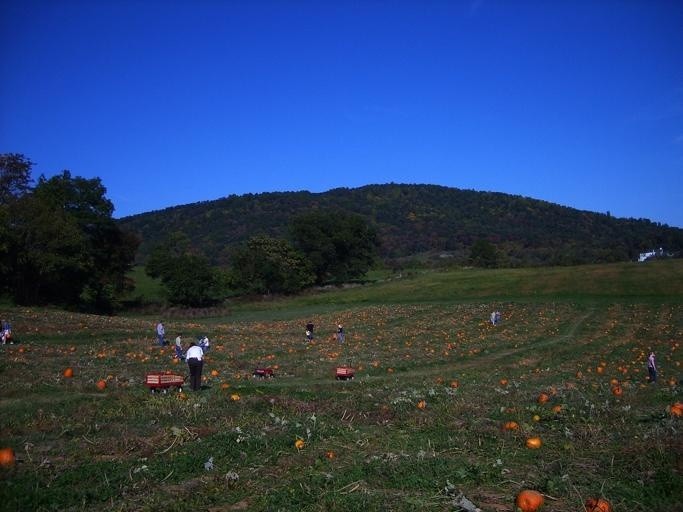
[145,371,185,387]
[255,368,273,375]
[336,366,355,377]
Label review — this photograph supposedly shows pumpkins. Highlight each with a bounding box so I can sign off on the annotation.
[0,303,682,512]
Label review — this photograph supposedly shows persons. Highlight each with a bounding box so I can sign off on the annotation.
[155,319,164,345]
[174,333,183,361]
[306,320,314,340]
[0,318,14,345]
[184,342,204,392]
[336,320,345,344]
[495,309,502,323]
[490,310,495,326]
[197,334,203,348]
[647,351,658,385]
[161,323,165,344]
[305,329,312,343]
[202,335,210,356]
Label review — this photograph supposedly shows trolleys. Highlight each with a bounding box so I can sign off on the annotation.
[334,360,355,381]
[145,372,191,393]
[254,368,275,377]
